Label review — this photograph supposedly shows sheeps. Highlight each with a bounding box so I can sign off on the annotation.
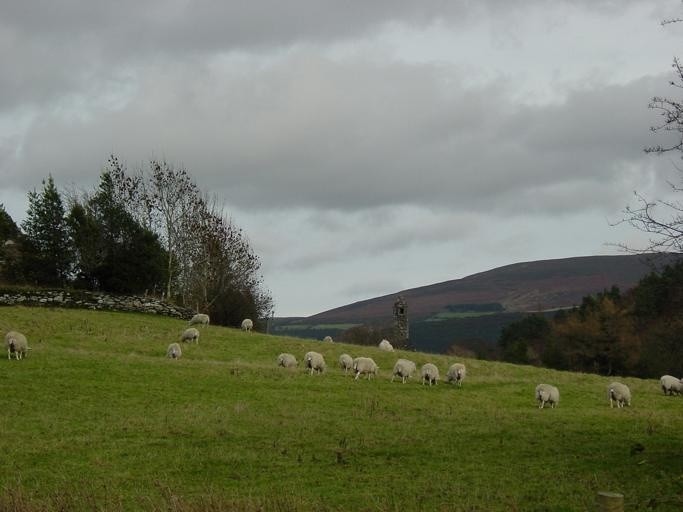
[276,351,326,377]
[535,383,560,410]
[166,343,182,361]
[378,339,394,351]
[390,358,466,388]
[607,382,631,409]
[4,331,32,361]
[240,319,254,332]
[188,314,210,328]
[660,375,683,396]
[181,328,200,346]
[338,353,381,382]
